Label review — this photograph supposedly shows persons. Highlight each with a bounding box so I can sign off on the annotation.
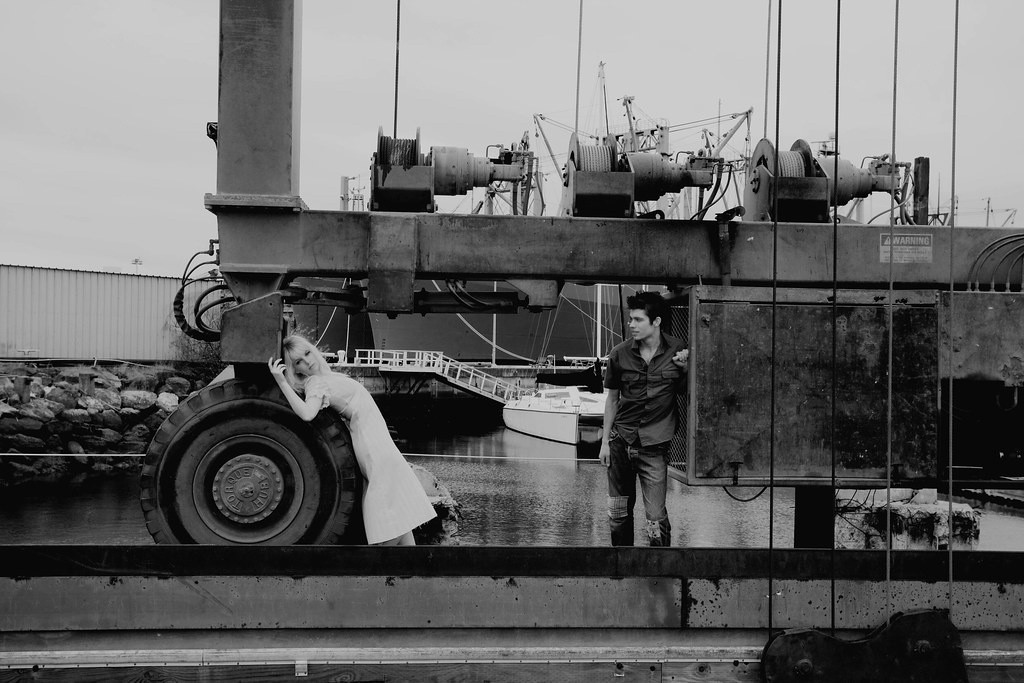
[269,337,437,545]
[599,291,691,545]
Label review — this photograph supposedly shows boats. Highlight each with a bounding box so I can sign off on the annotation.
[501,284,649,445]
